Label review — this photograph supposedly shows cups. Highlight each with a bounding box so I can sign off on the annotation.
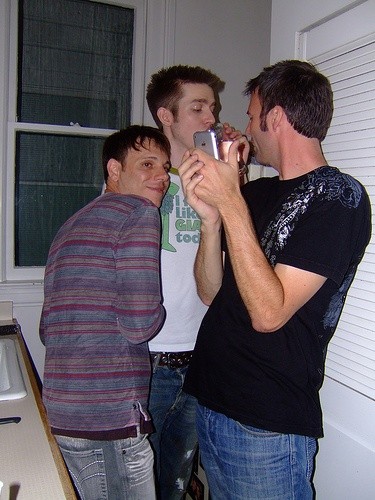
[207,122,248,179]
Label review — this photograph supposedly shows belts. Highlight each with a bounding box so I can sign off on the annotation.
[149,351,194,370]
[51,421,155,441]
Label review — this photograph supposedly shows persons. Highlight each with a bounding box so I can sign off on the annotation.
[177,59,372,500]
[38,125,171,500]
[101,64,251,500]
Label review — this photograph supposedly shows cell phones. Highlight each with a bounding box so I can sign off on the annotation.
[193,131,220,161]
